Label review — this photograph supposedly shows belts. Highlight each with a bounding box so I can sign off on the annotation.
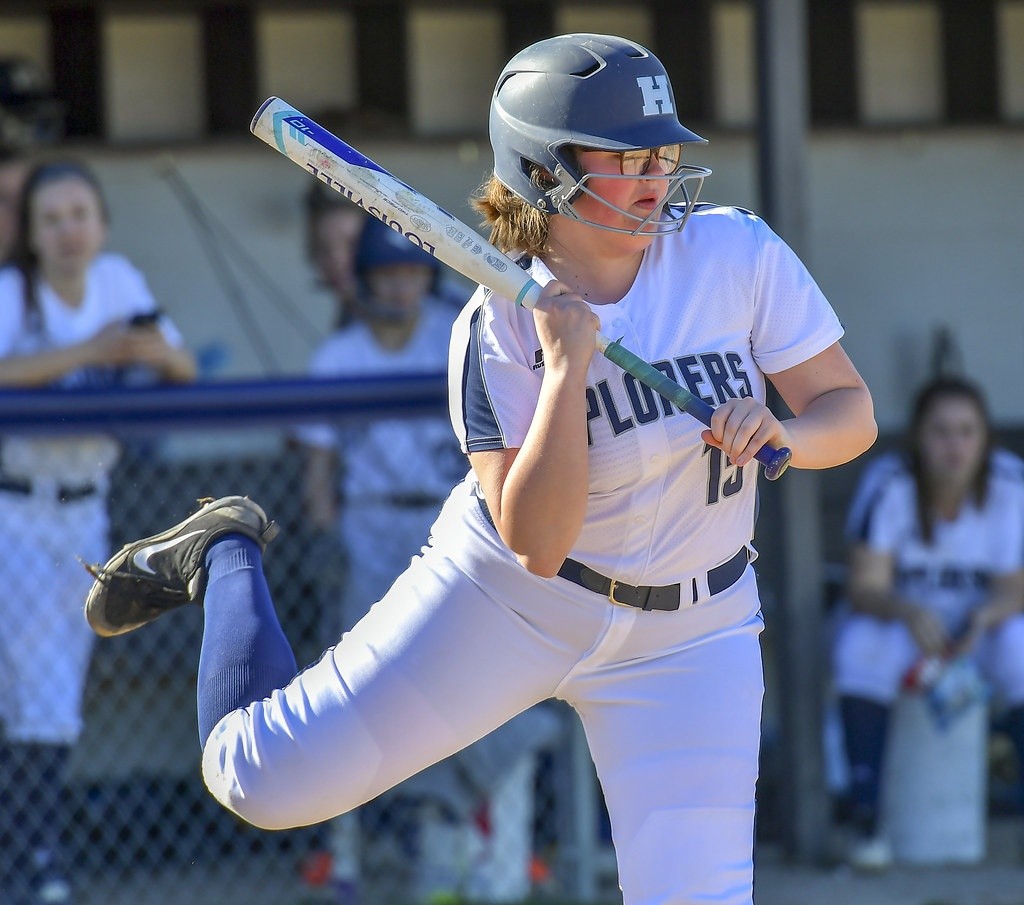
[466,480,755,614]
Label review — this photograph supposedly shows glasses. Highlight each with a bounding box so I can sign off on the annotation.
[570,138,683,180]
[928,421,988,444]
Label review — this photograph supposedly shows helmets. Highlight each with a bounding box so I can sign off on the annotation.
[354,212,444,292]
[489,33,709,212]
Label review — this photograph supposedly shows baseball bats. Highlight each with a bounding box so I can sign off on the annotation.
[248,85,793,491]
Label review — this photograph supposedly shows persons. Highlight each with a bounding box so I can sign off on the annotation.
[0,140,208,903]
[839,370,1023,869]
[276,160,543,897]
[78,33,876,904]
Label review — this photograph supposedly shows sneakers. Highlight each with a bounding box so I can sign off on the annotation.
[84,499,281,637]
[21,845,71,903]
[828,819,892,869]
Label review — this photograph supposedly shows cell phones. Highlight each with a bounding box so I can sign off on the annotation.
[131,312,159,325]
